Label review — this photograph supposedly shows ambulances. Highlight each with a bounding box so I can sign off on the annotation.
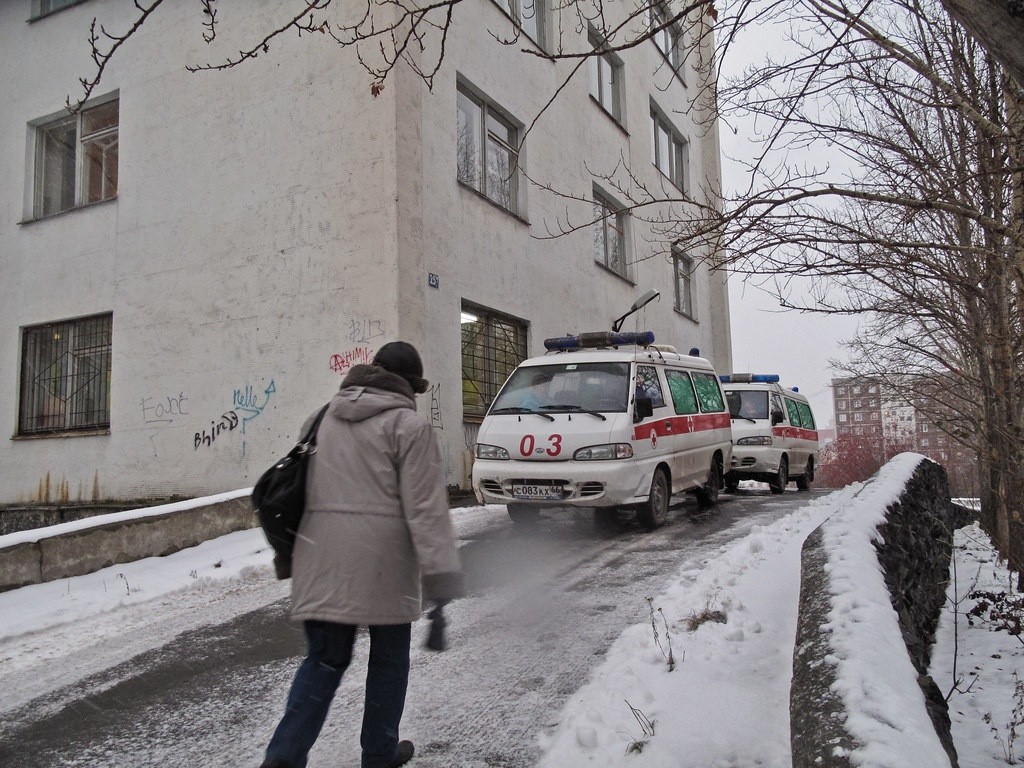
[470,332,734,527]
[716,373,819,493]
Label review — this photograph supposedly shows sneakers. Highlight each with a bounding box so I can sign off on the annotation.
[395,740,415,768]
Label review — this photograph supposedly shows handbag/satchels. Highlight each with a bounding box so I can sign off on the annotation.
[253,401,331,558]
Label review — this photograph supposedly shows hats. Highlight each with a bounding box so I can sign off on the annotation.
[371,341,430,395]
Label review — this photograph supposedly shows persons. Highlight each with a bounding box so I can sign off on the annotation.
[261,342,461,768]
[518,375,556,412]
